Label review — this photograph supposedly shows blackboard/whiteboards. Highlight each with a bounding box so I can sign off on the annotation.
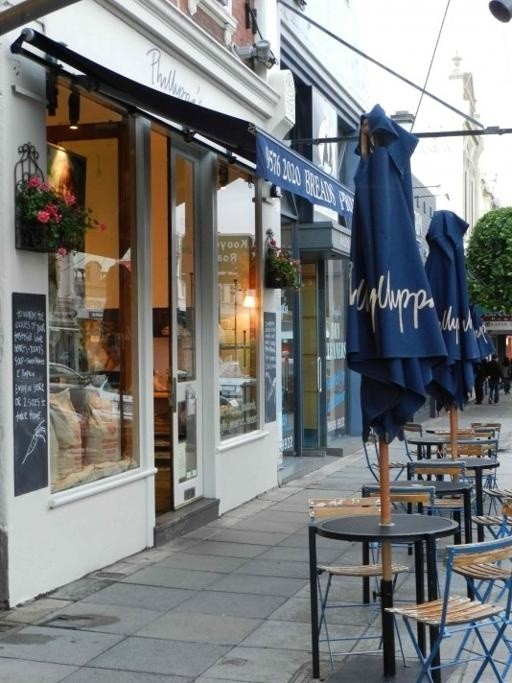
[264,311,276,424]
[12,292,48,497]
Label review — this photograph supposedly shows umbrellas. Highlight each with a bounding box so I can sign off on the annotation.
[344,104,448,525]
[425,210,481,462]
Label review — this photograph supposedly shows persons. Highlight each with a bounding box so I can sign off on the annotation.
[500,357,512,394]
[487,354,505,404]
[474,358,487,404]
[89,331,121,372]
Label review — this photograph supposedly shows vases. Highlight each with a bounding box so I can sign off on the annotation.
[24,221,84,250]
[267,276,287,288]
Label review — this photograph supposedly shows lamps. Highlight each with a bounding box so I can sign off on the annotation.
[68,82,80,130]
[236,41,275,69]
[243,292,256,308]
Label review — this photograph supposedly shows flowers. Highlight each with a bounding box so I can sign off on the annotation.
[16,176,108,256]
[266,240,306,289]
